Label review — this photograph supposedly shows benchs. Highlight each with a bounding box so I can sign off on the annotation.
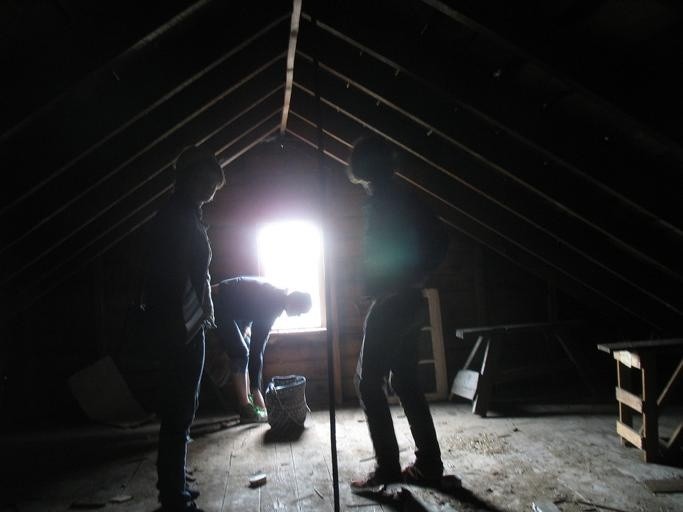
[449,319,582,415]
[597,338,683,463]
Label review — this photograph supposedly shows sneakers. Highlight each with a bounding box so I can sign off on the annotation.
[239,394,267,423]
[162,488,202,512]
[401,465,443,486]
[351,469,399,491]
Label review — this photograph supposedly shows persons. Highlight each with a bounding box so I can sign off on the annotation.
[212,276,312,424]
[143,146,227,512]
[351,137,445,499]
